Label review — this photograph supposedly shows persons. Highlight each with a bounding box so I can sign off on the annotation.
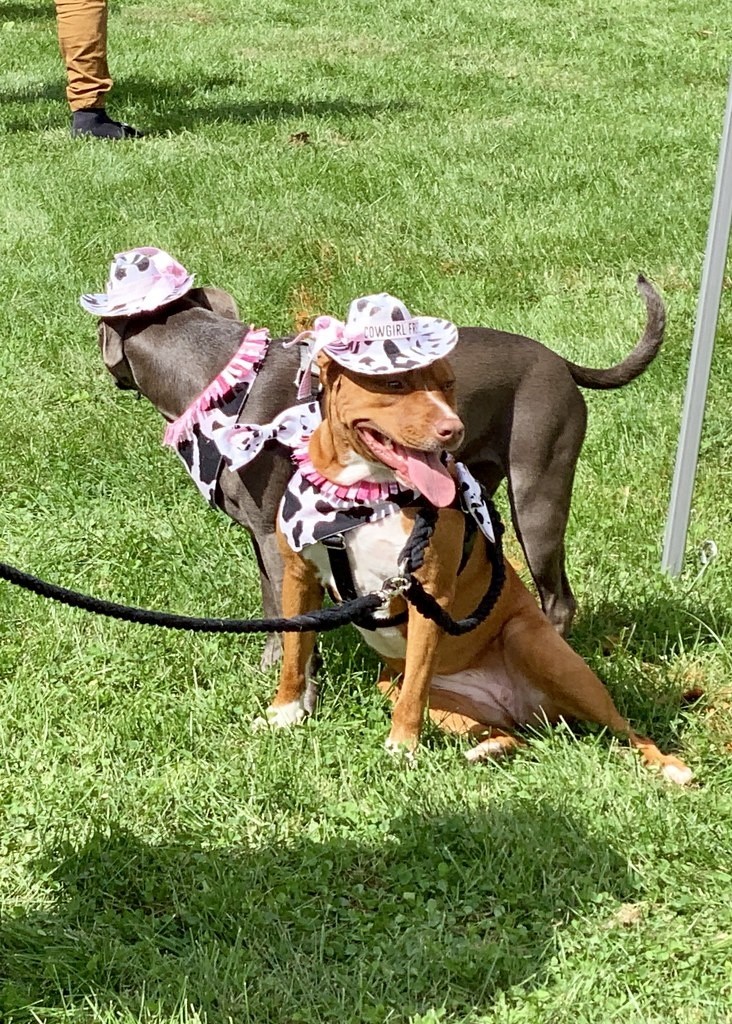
[54,0,141,140]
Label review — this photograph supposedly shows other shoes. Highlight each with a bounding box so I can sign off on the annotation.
[73,109,144,140]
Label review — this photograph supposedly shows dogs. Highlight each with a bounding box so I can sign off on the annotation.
[97,274,701,786]
